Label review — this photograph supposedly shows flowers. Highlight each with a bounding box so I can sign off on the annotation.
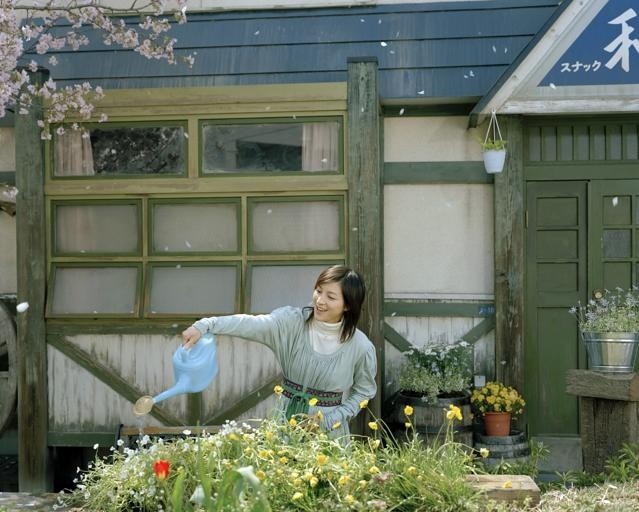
[394,339,527,413]
[569,282,639,332]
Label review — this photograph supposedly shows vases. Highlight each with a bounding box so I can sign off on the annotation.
[398,389,470,434]
[586,333,638,373]
[484,409,512,435]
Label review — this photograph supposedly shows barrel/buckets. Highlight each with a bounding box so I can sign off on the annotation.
[581,330,639,372]
[473,432,531,469]
[392,391,472,457]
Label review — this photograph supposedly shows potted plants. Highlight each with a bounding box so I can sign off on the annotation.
[479,138,509,174]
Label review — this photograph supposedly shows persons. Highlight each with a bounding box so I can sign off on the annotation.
[181,264,379,450]
